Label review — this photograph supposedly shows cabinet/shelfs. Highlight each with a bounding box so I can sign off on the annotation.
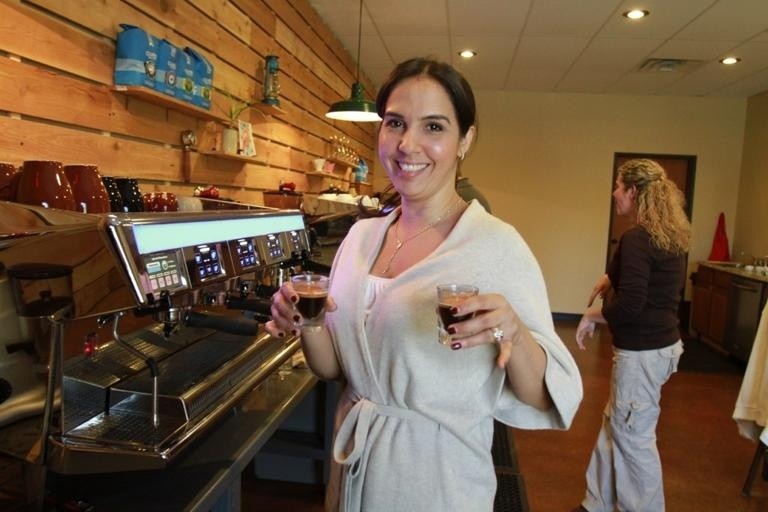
[304,157,372,186]
[103,84,272,166]
[688,264,735,349]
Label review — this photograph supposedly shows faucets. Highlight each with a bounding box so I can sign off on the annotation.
[740,251,759,266]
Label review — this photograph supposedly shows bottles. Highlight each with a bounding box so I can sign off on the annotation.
[328,135,360,165]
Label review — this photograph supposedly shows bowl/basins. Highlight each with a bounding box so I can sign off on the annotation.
[177,197,203,212]
[141,192,177,212]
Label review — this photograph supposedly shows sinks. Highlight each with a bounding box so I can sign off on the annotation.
[711,261,768,276]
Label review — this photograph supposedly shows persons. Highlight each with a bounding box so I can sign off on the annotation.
[260,57,588,511]
[450,156,496,214]
[575,158,695,511]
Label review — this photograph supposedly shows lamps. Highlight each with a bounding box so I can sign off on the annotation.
[325,0,384,122]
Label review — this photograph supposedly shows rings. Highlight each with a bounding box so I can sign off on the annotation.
[490,326,504,343]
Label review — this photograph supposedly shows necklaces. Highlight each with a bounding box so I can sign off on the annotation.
[382,192,465,275]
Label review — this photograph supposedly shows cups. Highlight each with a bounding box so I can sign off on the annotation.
[290,274,330,334]
[0,159,144,213]
[314,159,335,173]
[436,284,480,347]
[744,265,768,275]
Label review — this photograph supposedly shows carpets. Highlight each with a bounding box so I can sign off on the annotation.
[676,338,740,373]
[490,418,529,512]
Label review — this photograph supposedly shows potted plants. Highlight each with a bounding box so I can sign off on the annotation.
[211,89,262,156]
